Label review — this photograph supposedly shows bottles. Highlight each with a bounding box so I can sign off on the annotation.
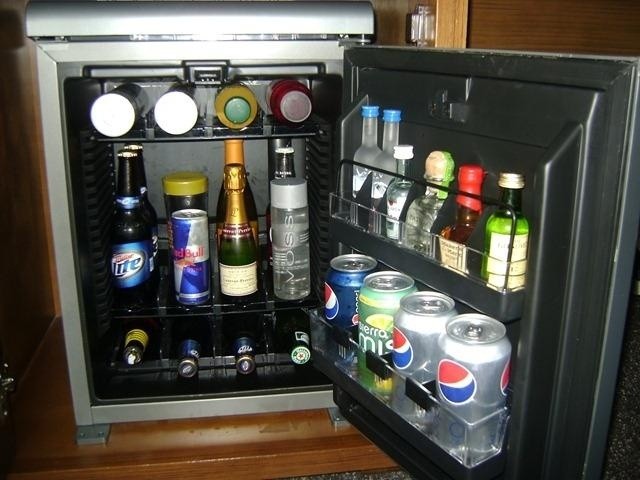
[171,209,209,306]
[170,316,212,377]
[217,163,258,302]
[404,151,456,260]
[162,171,209,274]
[436,313,512,453]
[266,147,296,266]
[124,146,159,289]
[216,139,258,262]
[265,79,314,130]
[271,177,310,301]
[275,303,311,365]
[479,173,530,294]
[358,271,422,397]
[215,82,258,131]
[324,254,378,366]
[392,292,459,421]
[441,165,490,276]
[223,314,261,376]
[352,106,383,231]
[387,146,423,243]
[110,153,154,312]
[370,110,404,236]
[122,318,162,366]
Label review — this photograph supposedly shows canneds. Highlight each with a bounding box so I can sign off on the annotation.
[154,89,200,135]
[90,83,149,137]
